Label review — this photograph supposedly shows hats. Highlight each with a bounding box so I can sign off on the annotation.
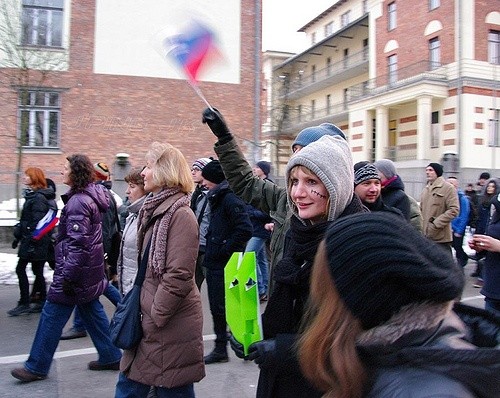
[92,162,110,181]
[354,161,381,191]
[256,161,270,177]
[427,163,444,177]
[193,157,213,171]
[324,212,465,331]
[373,159,397,179]
[480,172,490,179]
[202,160,225,184]
[291,123,346,153]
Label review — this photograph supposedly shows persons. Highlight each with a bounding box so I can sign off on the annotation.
[192,157,275,364]
[231,135,366,398]
[7,166,57,317]
[114,144,207,398]
[201,107,345,307]
[61,161,149,340]
[297,211,500,398]
[468,193,500,315]
[420,163,459,258]
[12,154,122,381]
[354,158,422,232]
[447,172,500,288]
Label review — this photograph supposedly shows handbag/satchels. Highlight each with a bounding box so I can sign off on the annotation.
[108,285,143,350]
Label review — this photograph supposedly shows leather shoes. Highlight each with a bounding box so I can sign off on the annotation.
[61,328,87,340]
[7,300,32,315]
[204,351,228,364]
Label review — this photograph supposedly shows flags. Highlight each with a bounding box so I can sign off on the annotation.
[167,24,210,83]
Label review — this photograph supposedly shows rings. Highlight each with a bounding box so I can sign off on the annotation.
[480,242,484,247]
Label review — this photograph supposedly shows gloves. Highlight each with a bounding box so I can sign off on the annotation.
[202,107,231,139]
[248,338,276,371]
[226,331,244,358]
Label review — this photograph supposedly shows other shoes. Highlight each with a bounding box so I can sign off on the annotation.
[30,301,45,312]
[89,361,120,370]
[9,366,47,381]
